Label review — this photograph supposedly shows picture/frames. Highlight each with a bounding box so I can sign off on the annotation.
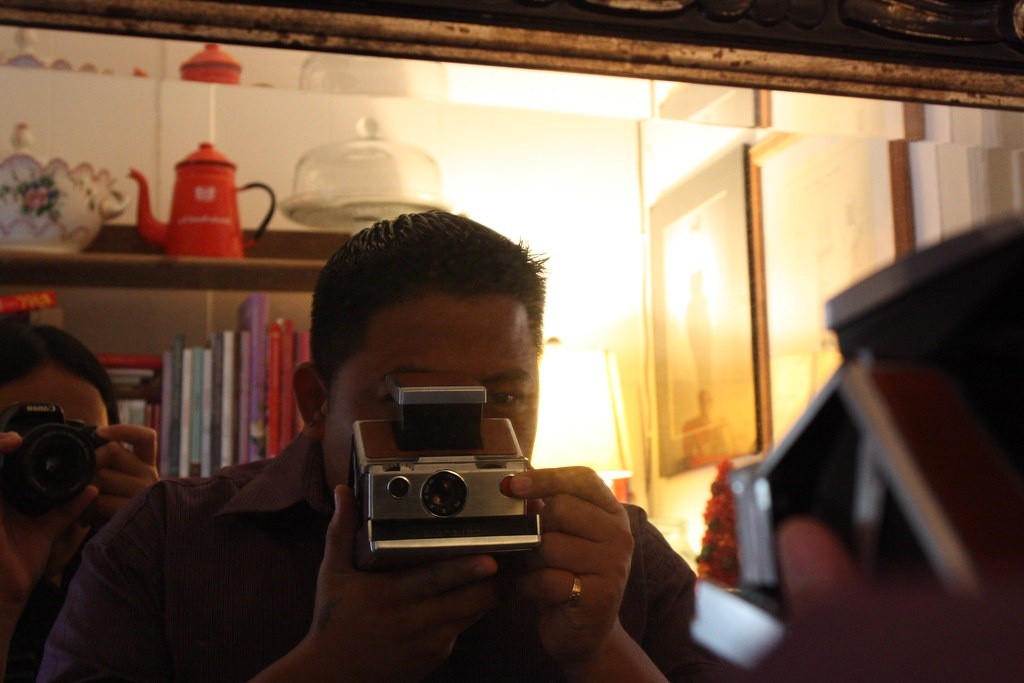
[646,144,772,478]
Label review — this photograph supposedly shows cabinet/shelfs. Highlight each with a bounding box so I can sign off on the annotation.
[0,209,366,498]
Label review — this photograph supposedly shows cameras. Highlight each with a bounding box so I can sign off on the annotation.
[0,400,105,514]
[350,387,542,555]
[731,218,1024,622]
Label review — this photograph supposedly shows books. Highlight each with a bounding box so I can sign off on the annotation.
[79,293,311,481]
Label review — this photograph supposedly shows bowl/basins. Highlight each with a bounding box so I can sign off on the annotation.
[282,118,452,226]
[0,155,128,252]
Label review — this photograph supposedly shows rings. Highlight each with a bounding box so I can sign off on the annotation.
[568,578,582,606]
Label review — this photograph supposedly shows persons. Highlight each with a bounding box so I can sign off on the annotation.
[0,323,161,683]
[37,209,708,683]
[745,214,1024,683]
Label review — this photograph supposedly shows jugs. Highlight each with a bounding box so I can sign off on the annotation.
[130,142,276,258]
